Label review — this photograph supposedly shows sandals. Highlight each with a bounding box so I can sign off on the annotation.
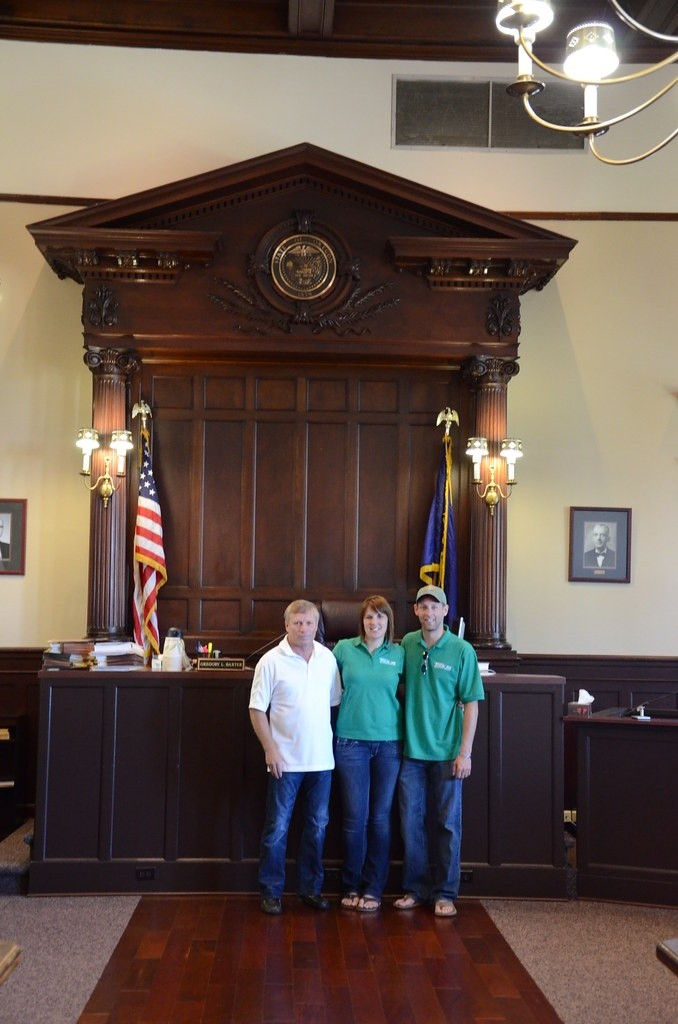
[357,896,381,912]
[434,897,457,917]
[394,896,422,909]
[341,894,359,908]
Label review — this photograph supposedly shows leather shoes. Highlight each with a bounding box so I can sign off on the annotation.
[298,893,329,910]
[261,897,282,913]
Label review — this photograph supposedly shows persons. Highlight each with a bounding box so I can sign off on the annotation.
[249,600,342,912]
[0,519,9,559]
[392,583,485,916]
[584,524,615,566]
[333,595,464,911]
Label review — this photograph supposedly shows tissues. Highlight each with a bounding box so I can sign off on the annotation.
[567,689,595,716]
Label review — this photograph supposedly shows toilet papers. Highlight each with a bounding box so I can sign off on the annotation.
[162,637,192,672]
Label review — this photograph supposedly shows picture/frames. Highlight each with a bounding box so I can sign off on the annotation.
[0,497,28,575]
[568,505,631,583]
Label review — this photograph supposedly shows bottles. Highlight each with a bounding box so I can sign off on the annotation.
[162,627,184,672]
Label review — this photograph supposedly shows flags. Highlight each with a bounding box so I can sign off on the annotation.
[131,432,167,666]
[420,436,457,628]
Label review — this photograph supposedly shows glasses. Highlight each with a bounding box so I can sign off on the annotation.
[421,650,429,675]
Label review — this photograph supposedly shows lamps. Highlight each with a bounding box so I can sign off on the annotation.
[75,425,133,508]
[465,438,522,516]
[495,1,678,164]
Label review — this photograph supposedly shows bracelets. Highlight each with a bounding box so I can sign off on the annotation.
[459,753,469,758]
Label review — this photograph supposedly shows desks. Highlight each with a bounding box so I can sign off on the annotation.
[563,714,678,909]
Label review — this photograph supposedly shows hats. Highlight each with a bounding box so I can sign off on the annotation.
[416,585,446,605]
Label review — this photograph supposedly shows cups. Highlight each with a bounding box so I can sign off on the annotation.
[50,642,62,654]
